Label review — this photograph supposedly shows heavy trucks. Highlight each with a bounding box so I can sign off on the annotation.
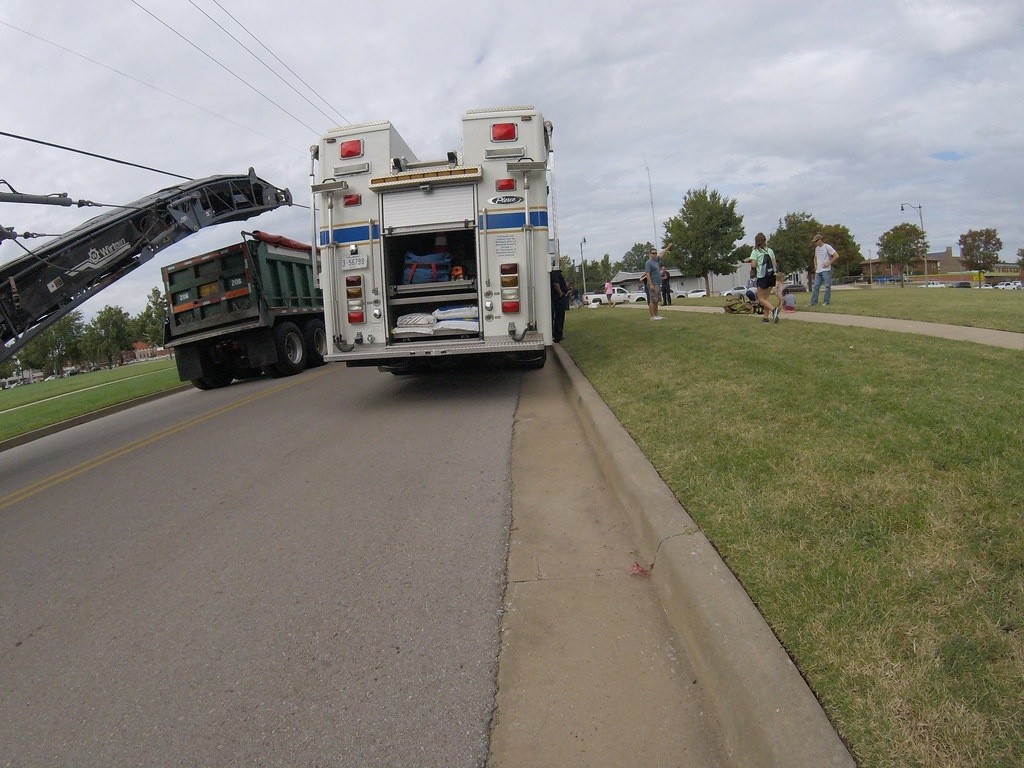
[160,231,328,392]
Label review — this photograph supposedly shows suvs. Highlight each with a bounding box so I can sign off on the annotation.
[948,281,971,288]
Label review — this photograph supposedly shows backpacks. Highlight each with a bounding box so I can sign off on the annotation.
[755,246,775,278]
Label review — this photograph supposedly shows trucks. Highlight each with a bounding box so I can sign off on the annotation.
[305,103,561,377]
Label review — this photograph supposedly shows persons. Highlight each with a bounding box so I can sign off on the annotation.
[550,259,567,340]
[661,267,671,307]
[804,234,839,307]
[604,278,616,308]
[567,284,579,310]
[749,232,780,323]
[782,289,796,313]
[640,274,650,305]
[746,286,757,301]
[645,243,674,320]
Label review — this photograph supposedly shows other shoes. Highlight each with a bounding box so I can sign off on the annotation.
[822,303,829,306]
[804,303,814,307]
[651,315,665,321]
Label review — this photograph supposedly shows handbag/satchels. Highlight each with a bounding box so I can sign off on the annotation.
[402,250,453,284]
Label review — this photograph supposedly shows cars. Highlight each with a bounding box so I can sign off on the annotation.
[719,285,747,296]
[688,288,707,299]
[994,281,1022,290]
[974,283,993,289]
[9,365,100,389]
[785,283,807,292]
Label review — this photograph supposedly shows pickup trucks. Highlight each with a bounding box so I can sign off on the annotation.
[582,286,630,304]
[630,284,689,303]
[919,281,946,288]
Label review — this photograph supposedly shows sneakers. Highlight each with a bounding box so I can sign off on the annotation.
[761,318,770,323]
[772,307,781,323]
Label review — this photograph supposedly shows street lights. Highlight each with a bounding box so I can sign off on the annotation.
[580,237,588,293]
[868,250,873,284]
[900,202,929,290]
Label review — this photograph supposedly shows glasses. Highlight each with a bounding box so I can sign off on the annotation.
[651,252,657,254]
[813,239,820,243]
